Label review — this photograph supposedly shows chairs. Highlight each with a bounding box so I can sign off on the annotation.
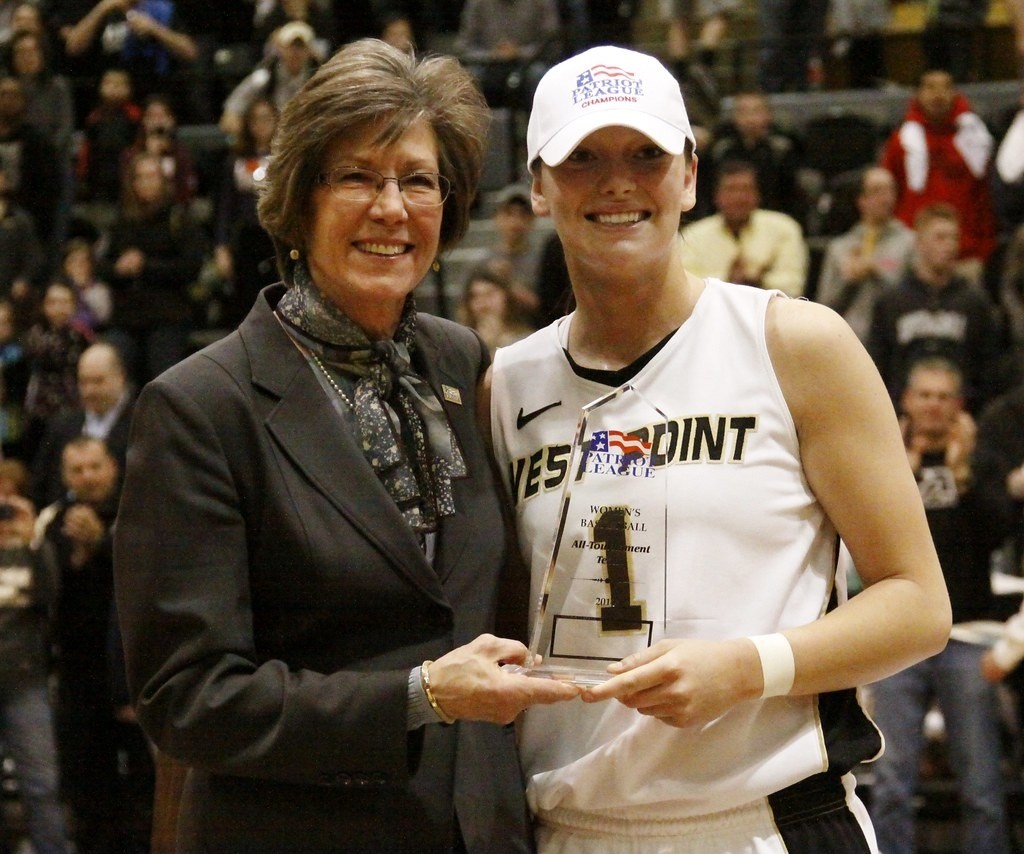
[414,103,562,332]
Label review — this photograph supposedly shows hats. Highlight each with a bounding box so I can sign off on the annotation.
[527,44,697,177]
[272,20,315,47]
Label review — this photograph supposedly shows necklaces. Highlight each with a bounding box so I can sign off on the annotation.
[308,349,357,411]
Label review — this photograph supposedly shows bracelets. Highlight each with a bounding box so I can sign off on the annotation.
[748,632,795,699]
[422,660,456,724]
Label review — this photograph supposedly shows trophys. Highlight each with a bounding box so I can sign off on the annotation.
[520,385,667,685]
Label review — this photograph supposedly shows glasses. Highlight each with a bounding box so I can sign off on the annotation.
[311,165,452,207]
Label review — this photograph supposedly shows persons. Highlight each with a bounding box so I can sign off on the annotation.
[115,38,585,854]
[0,0,1024,854]
[477,45,951,854]
